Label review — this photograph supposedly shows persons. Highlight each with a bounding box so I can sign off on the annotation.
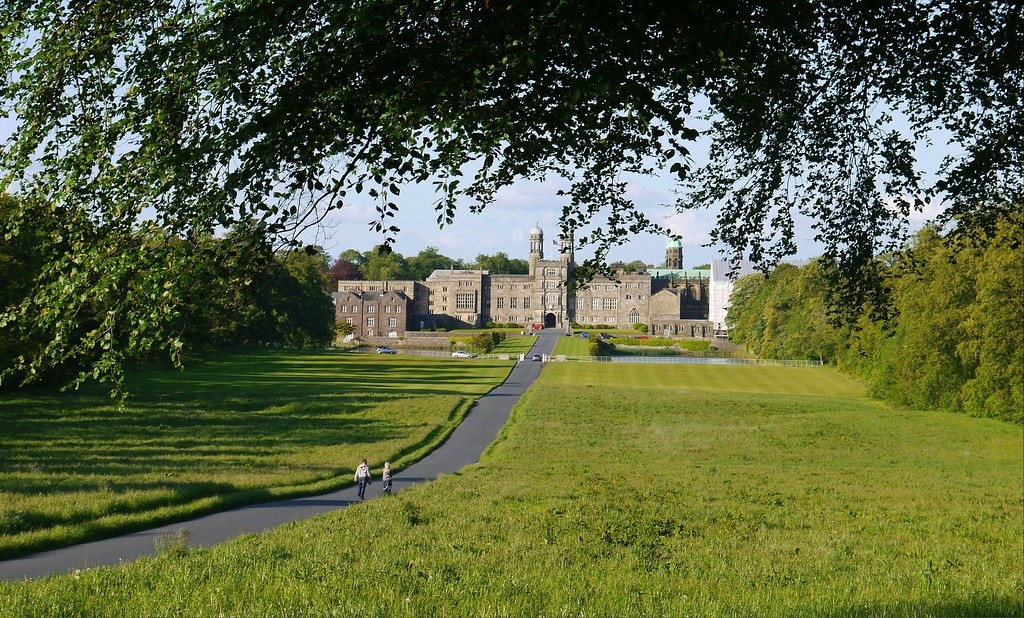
[354,459,373,500]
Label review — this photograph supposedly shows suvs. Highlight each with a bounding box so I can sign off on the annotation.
[377,346,396,354]
[600,333,613,339]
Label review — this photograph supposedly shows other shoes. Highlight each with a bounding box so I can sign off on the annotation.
[358,496,364,499]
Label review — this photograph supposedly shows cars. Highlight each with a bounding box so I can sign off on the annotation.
[579,333,589,338]
[634,336,648,339]
[533,324,543,330]
[452,351,472,359]
